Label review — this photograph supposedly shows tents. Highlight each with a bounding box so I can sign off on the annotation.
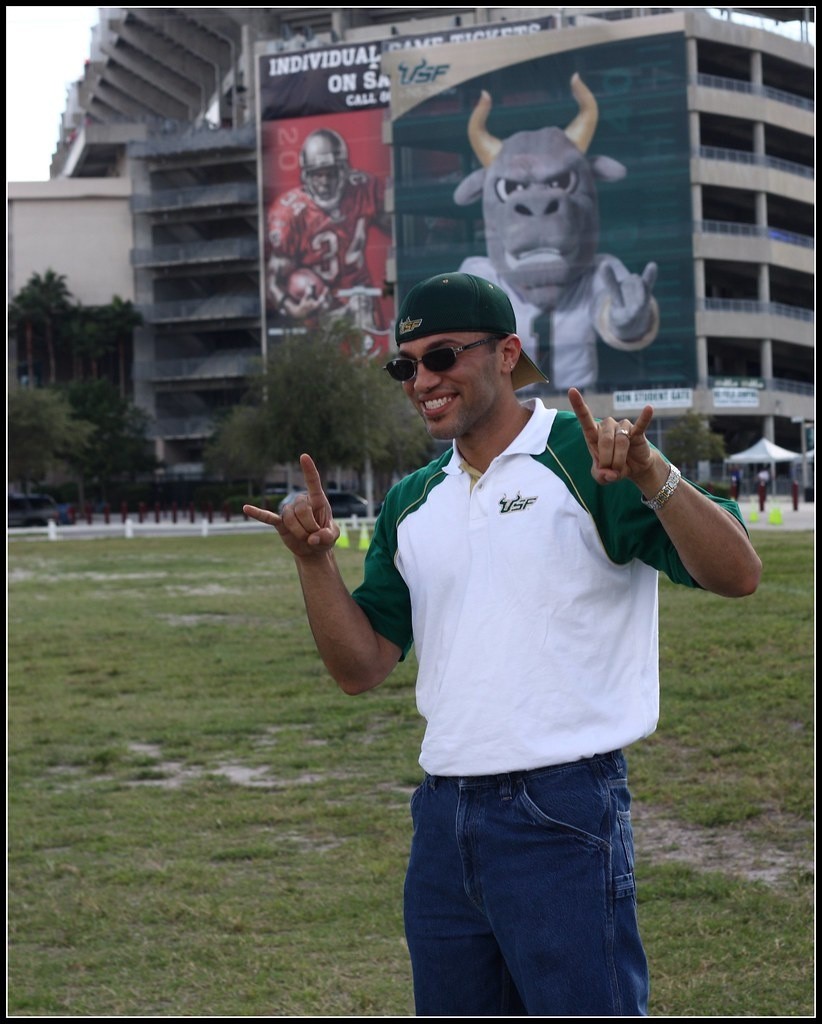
[724,438,803,495]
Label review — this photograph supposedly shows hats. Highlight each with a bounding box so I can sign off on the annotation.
[395,271,550,392]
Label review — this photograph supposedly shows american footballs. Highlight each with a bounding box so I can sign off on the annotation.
[287,267,327,306]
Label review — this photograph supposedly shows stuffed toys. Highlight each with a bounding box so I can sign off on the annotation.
[452,71,660,393]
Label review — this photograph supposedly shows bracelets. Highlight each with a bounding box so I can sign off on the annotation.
[641,464,681,511]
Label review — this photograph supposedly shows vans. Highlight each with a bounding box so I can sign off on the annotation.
[8,492,61,527]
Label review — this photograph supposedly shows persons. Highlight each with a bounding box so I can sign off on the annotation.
[759,466,769,482]
[730,466,740,485]
[266,128,391,363]
[240,272,762,1016]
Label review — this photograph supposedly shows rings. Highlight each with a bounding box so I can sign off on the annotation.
[616,430,630,439]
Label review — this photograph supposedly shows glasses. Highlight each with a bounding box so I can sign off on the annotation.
[383,334,508,382]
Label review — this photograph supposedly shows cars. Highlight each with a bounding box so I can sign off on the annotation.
[264,480,383,519]
